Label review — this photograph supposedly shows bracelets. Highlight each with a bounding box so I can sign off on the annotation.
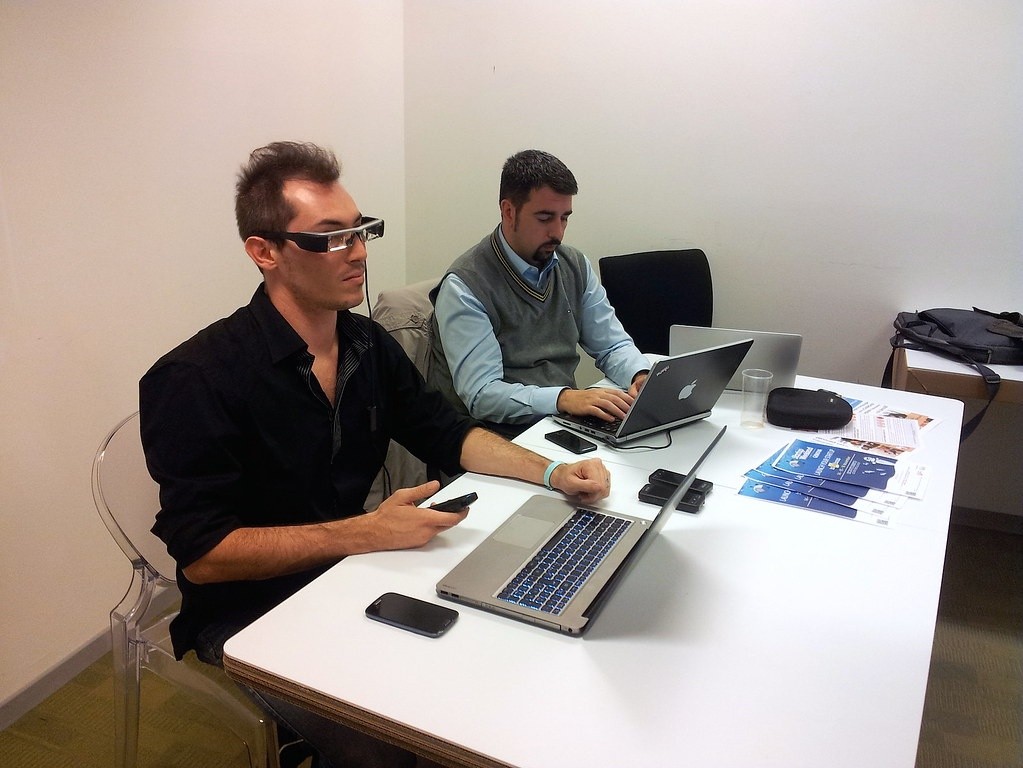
[543,460,567,490]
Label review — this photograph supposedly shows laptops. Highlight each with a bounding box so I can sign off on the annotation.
[435,425,727,636]
[669,325,802,393]
[552,338,753,444]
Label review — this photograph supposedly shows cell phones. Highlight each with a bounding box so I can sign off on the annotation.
[649,469,713,496]
[545,430,597,455]
[638,484,706,514]
[364,592,459,638]
[428,492,479,513]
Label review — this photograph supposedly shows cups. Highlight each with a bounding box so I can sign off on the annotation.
[741,368,773,429]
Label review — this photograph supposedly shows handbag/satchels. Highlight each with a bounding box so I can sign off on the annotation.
[893,307,1023,367]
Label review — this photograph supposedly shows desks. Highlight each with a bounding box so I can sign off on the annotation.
[891,338,1023,535]
[223,351,963,768]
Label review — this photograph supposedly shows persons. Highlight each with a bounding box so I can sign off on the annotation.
[141,142,611,767]
[424,148,654,492]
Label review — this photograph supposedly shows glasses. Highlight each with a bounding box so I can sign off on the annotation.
[254,217,385,254]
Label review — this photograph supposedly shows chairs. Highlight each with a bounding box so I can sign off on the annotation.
[364,273,442,516]
[90,408,283,768]
[599,248,712,356]
